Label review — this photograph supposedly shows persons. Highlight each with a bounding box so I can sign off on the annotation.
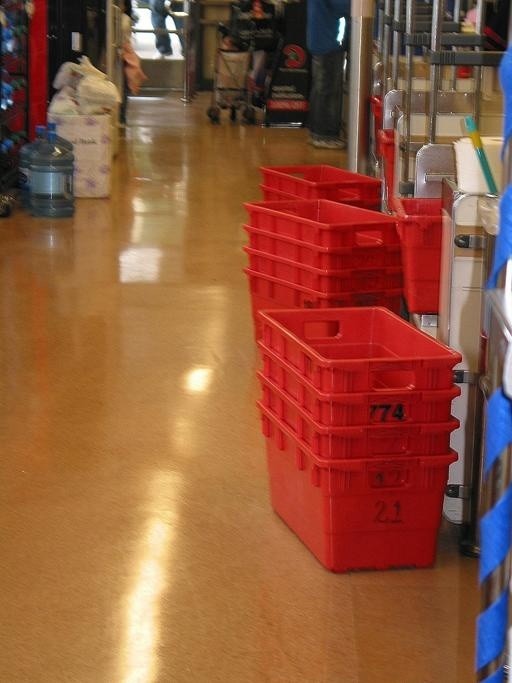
[151,1,184,61]
[304,0,351,151]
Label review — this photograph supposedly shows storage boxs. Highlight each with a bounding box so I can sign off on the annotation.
[46,112,111,201]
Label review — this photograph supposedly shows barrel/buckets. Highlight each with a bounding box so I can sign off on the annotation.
[44,123,75,152]
[15,125,46,210]
[28,131,75,219]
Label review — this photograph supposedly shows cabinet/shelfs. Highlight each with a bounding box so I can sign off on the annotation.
[0,0,47,192]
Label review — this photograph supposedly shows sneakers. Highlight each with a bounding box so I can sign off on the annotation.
[308,133,348,150]
